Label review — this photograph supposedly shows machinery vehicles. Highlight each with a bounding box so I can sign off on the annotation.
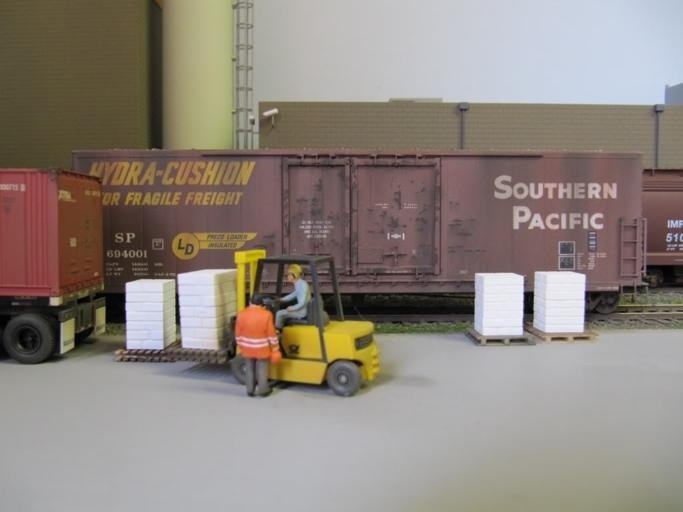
[114,247,380,397]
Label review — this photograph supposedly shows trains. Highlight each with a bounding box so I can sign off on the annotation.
[73,148,683,315]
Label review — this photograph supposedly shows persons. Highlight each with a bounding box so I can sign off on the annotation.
[233,294,282,398]
[273,263,313,334]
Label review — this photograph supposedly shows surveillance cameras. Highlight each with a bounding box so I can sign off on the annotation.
[262,106,279,126]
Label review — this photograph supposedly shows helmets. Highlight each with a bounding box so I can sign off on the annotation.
[287,264,302,279]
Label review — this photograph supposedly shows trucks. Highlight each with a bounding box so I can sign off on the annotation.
[0,168,105,362]
